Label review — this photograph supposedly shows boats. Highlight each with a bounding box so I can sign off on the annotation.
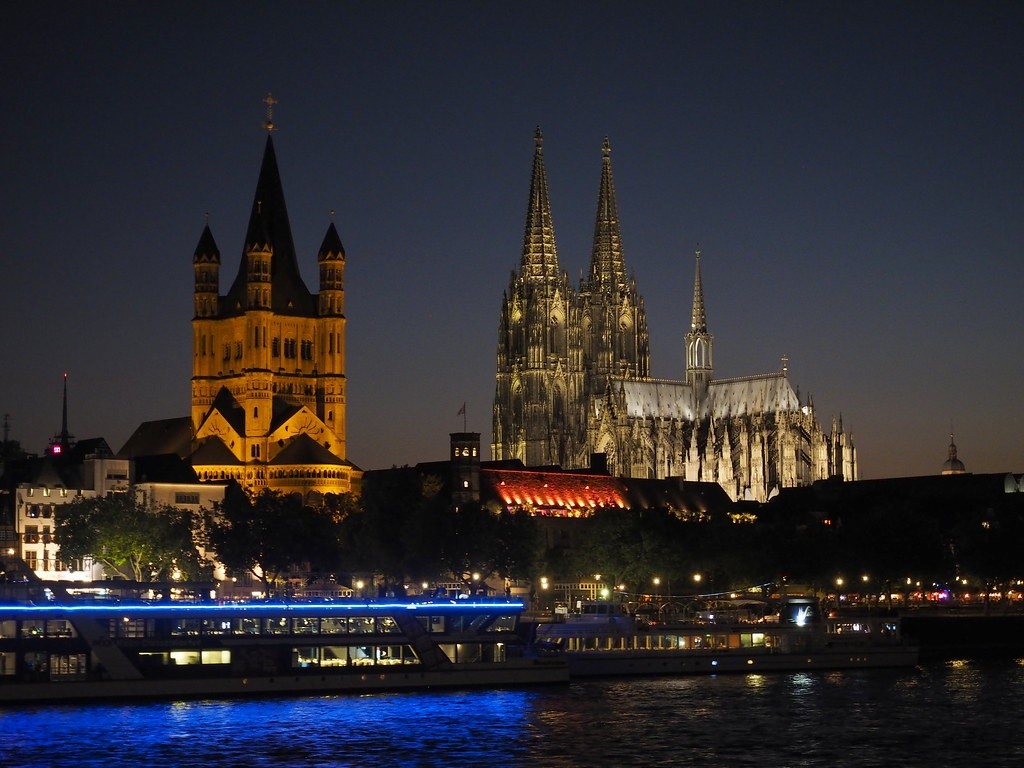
[546,595,922,673]
